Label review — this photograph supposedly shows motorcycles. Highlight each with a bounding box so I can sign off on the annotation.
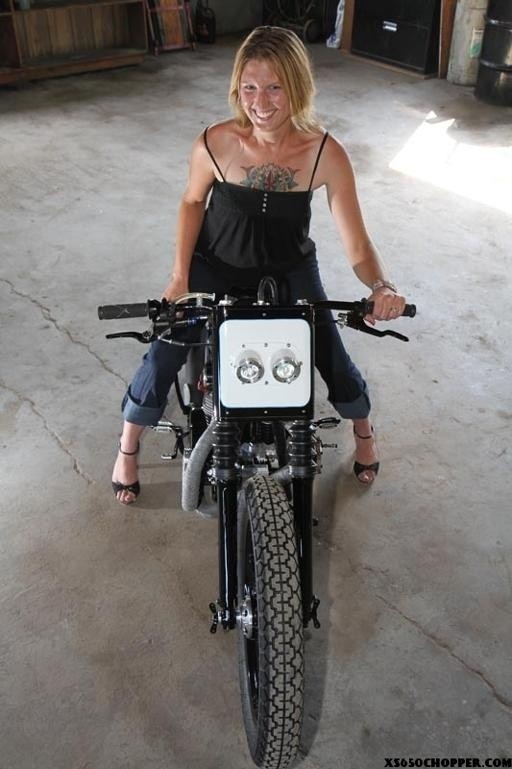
[98,278,417,764]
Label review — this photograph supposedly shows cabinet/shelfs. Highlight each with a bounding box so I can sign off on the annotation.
[351,0,441,74]
[0,0,149,87]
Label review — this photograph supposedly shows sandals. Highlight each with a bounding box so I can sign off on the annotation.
[352,423,380,487]
[110,438,142,507]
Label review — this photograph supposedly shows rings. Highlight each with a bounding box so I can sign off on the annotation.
[392,307,399,313]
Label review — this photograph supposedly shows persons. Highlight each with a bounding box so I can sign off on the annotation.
[111,24,407,508]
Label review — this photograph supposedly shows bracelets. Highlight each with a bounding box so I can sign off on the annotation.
[371,278,400,294]
